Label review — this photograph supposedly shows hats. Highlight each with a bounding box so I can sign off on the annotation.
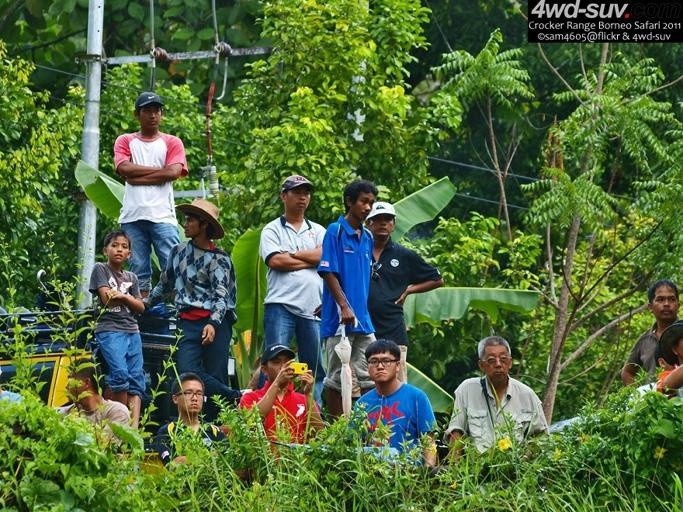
[135,92,165,108]
[176,199,225,240]
[260,343,296,364]
[366,201,398,219]
[659,322,682,366]
[282,175,314,193]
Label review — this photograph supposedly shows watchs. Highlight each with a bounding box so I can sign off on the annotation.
[288,250,297,256]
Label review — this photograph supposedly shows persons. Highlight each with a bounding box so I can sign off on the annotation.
[444,336,549,464]
[114,92,188,298]
[240,343,324,460]
[317,181,377,424]
[347,338,438,467]
[621,279,683,400]
[0,389,22,403]
[365,201,444,384]
[55,357,131,448]
[89,229,145,429]
[144,198,253,427]
[259,174,327,409]
[155,372,230,473]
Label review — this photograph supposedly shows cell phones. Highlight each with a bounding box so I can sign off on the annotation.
[289,362,308,374]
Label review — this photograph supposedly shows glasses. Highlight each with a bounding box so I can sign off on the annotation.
[368,358,398,366]
[175,390,203,400]
[370,262,382,282]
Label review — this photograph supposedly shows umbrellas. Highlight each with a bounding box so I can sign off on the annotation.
[334,316,358,419]
